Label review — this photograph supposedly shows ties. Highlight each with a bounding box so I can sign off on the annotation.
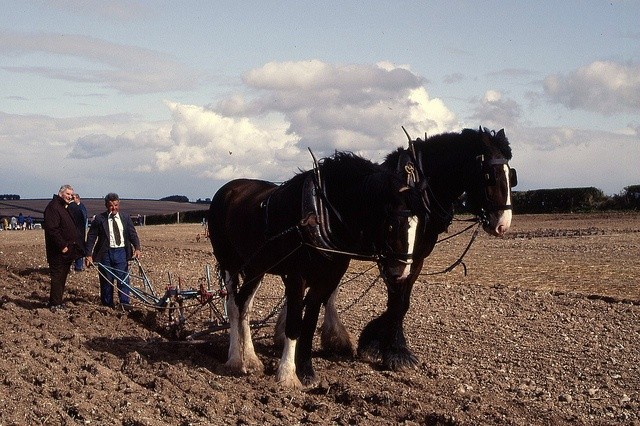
[111,216,121,246]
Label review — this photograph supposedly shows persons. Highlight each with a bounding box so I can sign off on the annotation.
[43,184,80,310]
[10,215,17,230]
[135,212,144,229]
[85,192,141,311]
[3,218,9,231]
[26,215,32,230]
[17,212,25,230]
[92,213,96,223]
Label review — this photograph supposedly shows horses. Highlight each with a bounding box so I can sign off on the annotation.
[207,146,431,390]
[274,127,513,372]
